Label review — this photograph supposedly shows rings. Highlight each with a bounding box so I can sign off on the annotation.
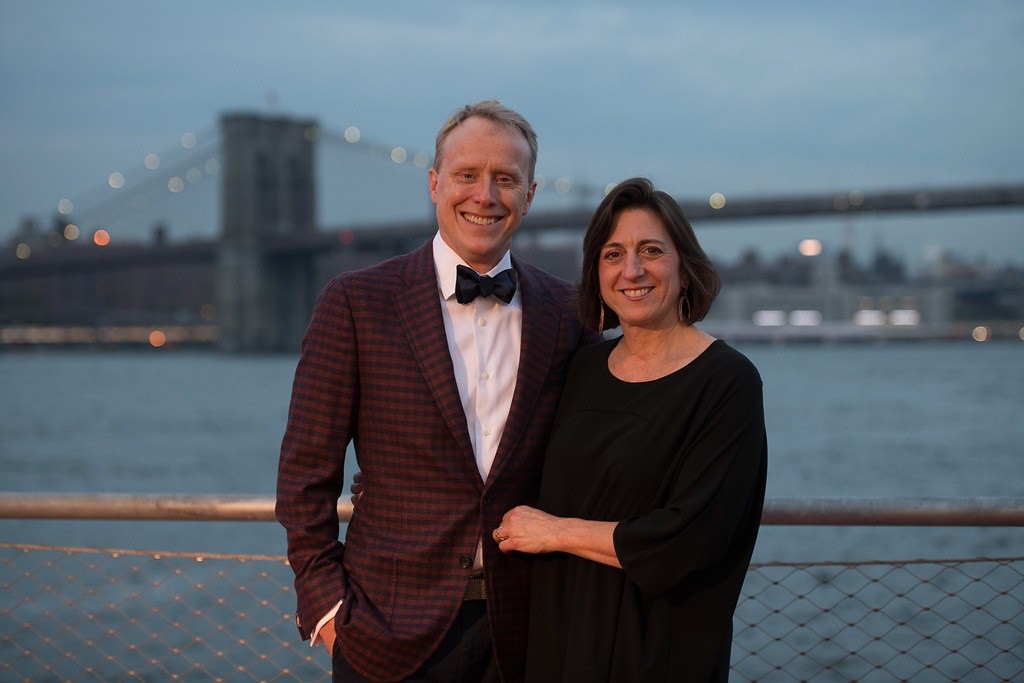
[494,527,508,541]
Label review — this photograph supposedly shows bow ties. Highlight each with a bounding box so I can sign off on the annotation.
[455,265,518,304]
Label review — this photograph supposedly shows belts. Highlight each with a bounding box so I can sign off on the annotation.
[465,578,487,601]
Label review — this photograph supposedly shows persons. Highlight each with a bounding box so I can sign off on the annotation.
[351,177,768,682]
[275,100,606,683]
